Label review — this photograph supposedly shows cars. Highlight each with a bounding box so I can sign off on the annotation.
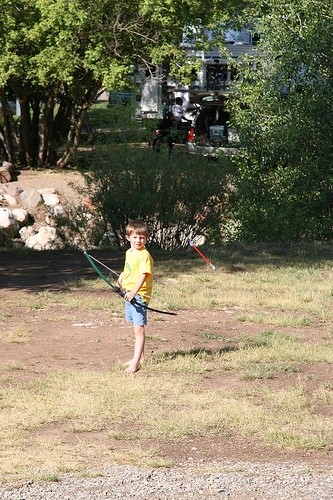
[12,113,78,156]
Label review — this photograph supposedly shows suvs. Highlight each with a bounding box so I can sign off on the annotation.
[188,93,253,158]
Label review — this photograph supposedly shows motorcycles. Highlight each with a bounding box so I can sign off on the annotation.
[151,103,200,155]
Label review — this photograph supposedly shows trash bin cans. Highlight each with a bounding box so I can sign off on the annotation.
[209,126,224,140]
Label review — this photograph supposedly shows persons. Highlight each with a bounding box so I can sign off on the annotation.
[115,220,154,373]
[171,97,189,123]
